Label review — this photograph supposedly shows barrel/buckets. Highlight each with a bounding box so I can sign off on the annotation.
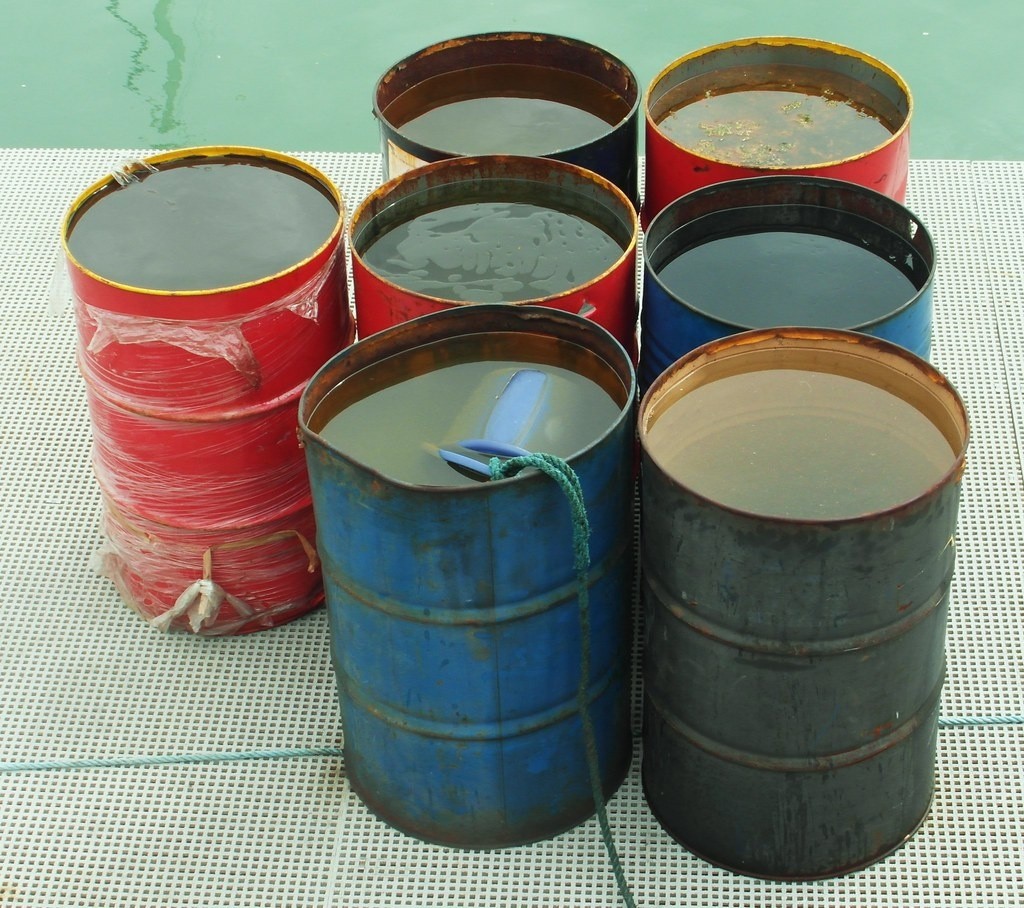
[642,37,913,245]
[349,152,637,358]
[639,176,935,409]
[298,302,633,851]
[62,146,356,636]
[372,32,639,204]
[636,325,970,882]
